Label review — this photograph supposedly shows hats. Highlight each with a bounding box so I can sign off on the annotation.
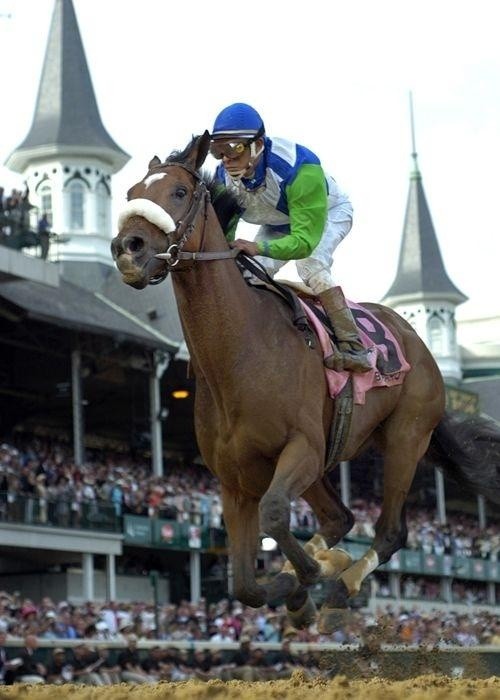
[211,102,267,139]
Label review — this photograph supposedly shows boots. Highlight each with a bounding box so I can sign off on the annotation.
[316,284,373,374]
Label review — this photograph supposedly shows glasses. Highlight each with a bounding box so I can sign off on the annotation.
[209,122,265,160]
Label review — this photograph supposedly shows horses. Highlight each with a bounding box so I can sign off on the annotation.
[110,128,500,636]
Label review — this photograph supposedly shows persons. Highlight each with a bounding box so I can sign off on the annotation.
[0,439,499,561]
[0,180,52,259]
[211,103,373,372]
[0,592,499,691]
